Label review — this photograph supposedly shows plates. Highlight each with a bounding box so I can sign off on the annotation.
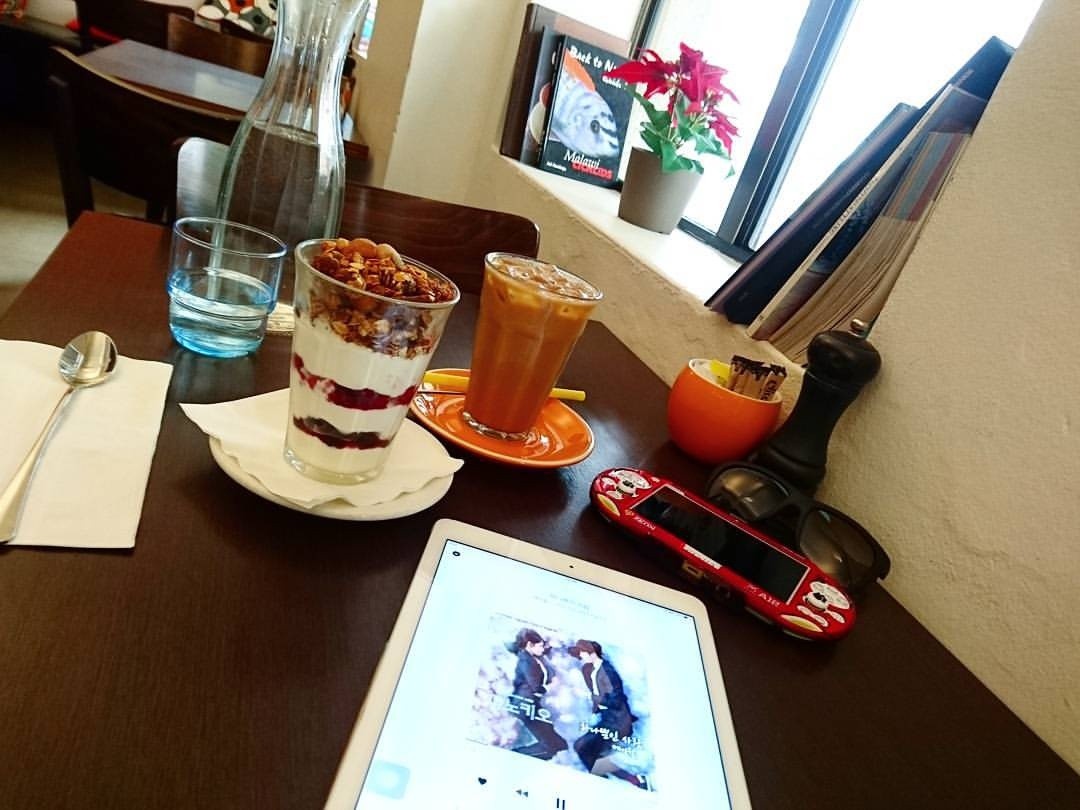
[410,368,595,468]
[208,417,456,520]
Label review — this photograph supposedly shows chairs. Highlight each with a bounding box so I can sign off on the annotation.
[45,0,540,295]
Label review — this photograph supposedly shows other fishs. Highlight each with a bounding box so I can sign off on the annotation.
[551,48,620,159]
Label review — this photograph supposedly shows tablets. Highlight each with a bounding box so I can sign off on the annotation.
[325,518,753,810]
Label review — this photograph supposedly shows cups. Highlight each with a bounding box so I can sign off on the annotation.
[666,358,783,465]
[284,238,461,486]
[459,252,605,442]
[164,217,288,360]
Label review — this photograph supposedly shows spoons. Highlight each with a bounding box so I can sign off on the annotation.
[1,330,119,542]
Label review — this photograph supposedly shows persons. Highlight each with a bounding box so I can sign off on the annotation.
[510,629,568,760]
[568,639,650,792]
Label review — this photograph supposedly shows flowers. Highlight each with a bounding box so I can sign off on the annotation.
[604,40,741,179]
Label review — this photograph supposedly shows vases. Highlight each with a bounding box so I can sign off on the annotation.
[619,146,700,236]
[208,0,367,334]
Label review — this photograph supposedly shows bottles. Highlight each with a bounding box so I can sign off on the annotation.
[207,0,373,336]
[760,316,882,496]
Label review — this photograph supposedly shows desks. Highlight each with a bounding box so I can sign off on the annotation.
[75,39,370,180]
[0,210,1080,810]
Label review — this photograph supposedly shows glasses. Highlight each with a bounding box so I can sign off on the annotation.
[703,462,891,597]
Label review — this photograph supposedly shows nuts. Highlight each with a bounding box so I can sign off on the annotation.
[310,237,451,357]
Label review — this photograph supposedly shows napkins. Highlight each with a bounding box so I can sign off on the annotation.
[0,340,174,548]
[179,388,465,508]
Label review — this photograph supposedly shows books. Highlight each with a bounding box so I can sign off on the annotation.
[704,35,1015,368]
[500,4,637,189]
